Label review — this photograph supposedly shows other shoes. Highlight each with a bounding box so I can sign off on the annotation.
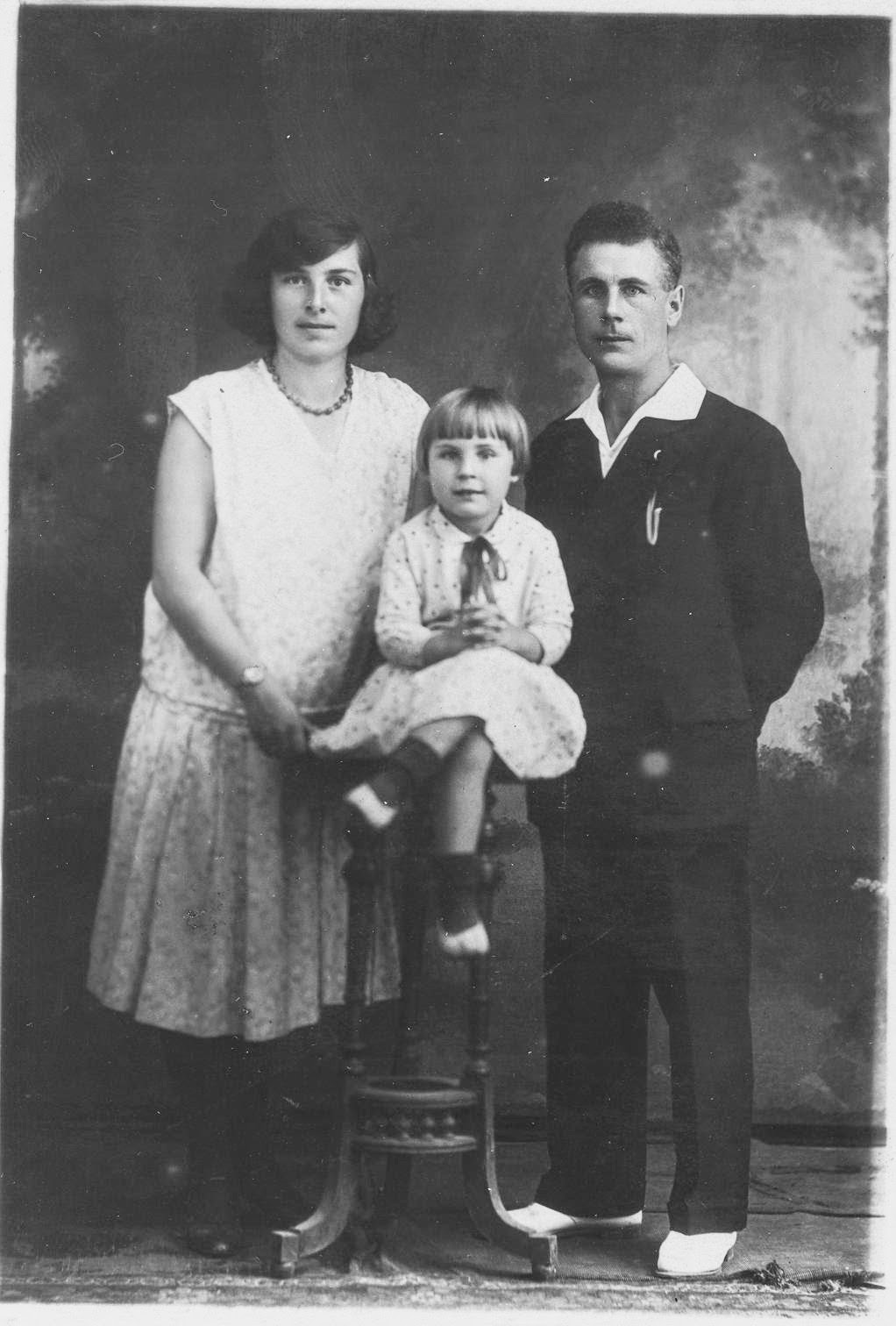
[172,1198,237,1259]
[434,911,490,960]
[347,783,399,829]
[653,1229,738,1282]
[503,1198,642,1240]
[258,1185,311,1236]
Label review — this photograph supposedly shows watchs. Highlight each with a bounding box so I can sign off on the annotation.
[234,659,270,693]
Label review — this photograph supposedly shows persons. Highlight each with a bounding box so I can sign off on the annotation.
[507,198,825,1280]
[306,385,588,958]
[86,206,438,1259]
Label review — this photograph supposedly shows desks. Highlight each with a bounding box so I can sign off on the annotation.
[270,713,560,1282]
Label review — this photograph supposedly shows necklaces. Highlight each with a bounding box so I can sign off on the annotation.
[263,347,353,417]
